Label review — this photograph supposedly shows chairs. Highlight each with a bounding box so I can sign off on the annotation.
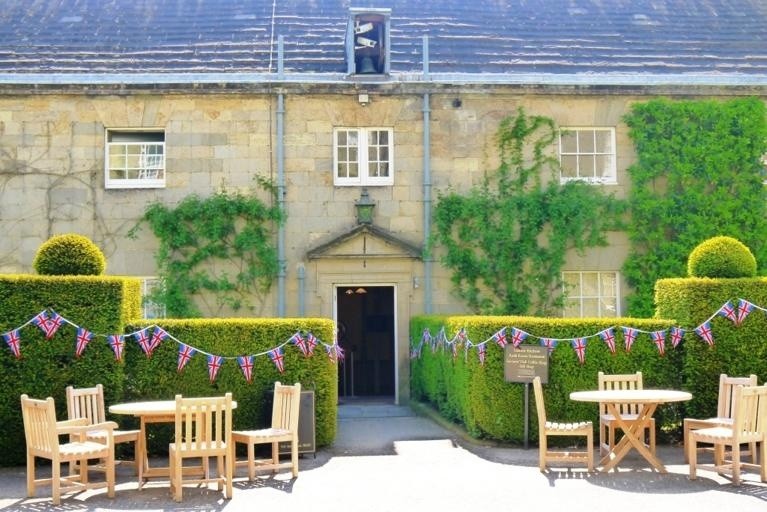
[531,375,594,474]
[16,380,303,504]
[595,369,766,486]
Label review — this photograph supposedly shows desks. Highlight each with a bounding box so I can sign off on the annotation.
[569,388,692,477]
